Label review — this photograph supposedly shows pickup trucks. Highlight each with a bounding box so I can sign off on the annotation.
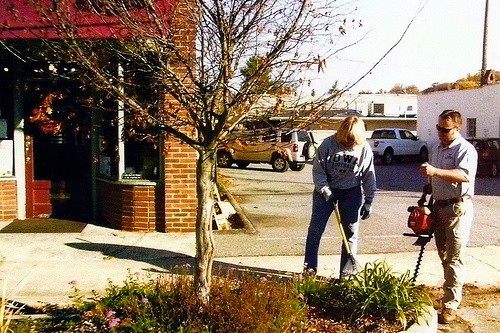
[365,128,429,164]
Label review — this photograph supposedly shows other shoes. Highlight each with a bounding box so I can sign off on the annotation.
[439,308,456,324]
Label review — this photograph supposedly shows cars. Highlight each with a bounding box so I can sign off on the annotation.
[466,137,500,179]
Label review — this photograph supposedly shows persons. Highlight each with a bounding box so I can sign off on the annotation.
[304,115,376,283]
[419,111,477,323]
[51,99,74,194]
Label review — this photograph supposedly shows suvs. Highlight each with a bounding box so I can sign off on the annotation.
[217,117,318,172]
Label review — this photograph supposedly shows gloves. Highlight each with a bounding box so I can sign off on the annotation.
[323,188,337,211]
[360,199,372,220]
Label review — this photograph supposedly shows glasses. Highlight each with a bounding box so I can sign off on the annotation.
[436,123,458,133]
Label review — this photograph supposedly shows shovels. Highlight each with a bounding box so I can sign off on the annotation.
[309,132,363,279]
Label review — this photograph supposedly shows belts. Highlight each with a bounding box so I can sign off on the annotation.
[435,194,472,207]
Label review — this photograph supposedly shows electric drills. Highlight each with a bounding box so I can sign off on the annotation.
[402,183,437,287]
